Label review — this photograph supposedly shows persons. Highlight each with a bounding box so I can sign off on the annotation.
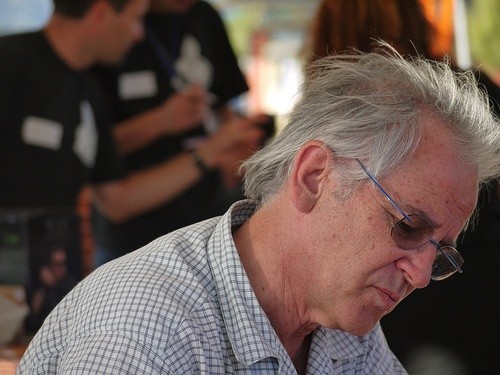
[15,37,500,375]
[0,0,275,349]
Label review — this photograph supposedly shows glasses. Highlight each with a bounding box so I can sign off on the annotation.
[355,158,464,280]
